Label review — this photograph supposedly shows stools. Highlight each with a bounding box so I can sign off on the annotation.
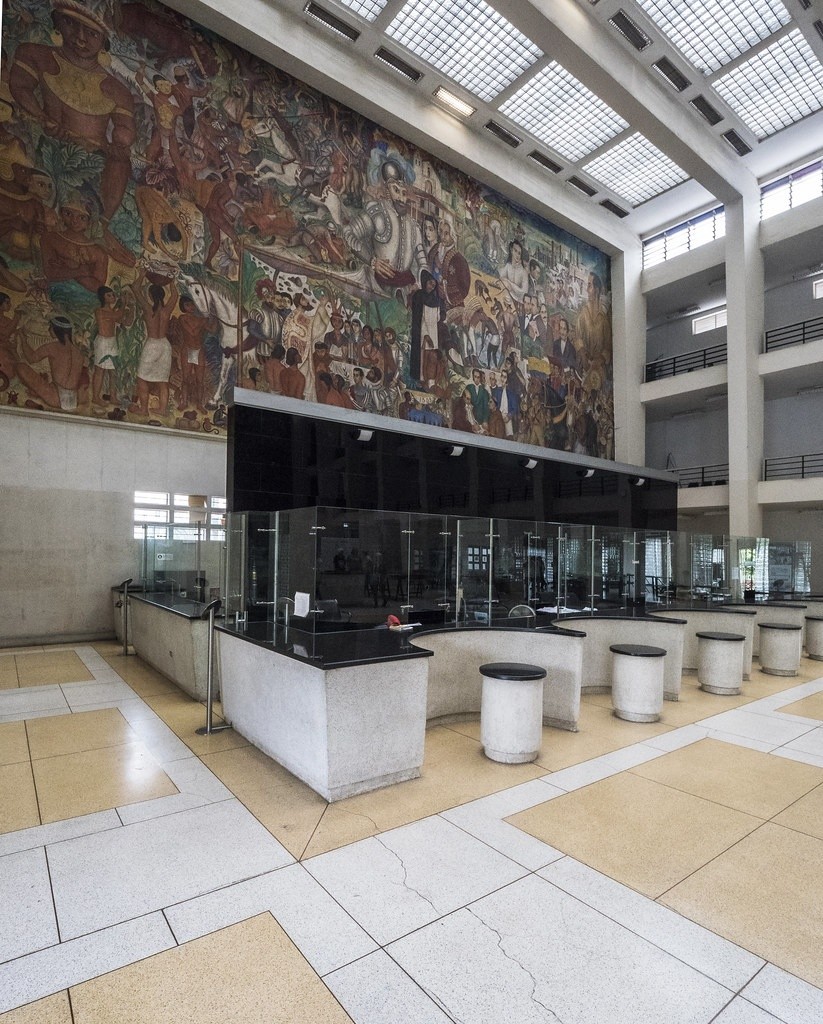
[696,632,746,695]
[479,664,547,764]
[757,622,802,676]
[609,644,667,723]
[805,616,823,662]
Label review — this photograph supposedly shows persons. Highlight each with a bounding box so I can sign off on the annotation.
[333,547,388,608]
[522,556,547,601]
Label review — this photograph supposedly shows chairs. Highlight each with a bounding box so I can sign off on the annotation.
[701,481,713,486]
[714,479,726,485]
[687,482,699,487]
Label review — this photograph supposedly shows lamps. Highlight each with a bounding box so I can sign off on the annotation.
[582,469,595,478]
[522,458,538,470]
[351,428,376,442]
[633,477,649,489]
[447,445,465,456]
